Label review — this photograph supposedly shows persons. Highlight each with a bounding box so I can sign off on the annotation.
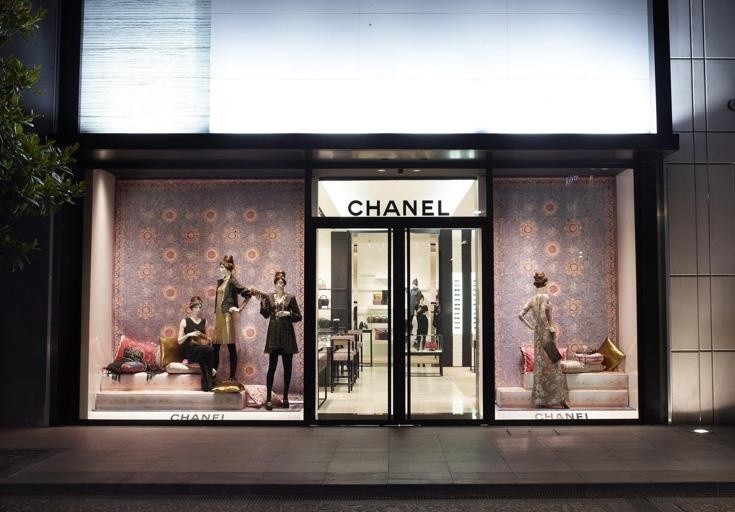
[432,295,442,335]
[249,270,302,411]
[212,255,252,388]
[519,272,571,410]
[416,293,428,352]
[178,296,214,392]
[410,277,421,337]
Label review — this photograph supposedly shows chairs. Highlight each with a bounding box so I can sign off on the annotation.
[330,328,365,394]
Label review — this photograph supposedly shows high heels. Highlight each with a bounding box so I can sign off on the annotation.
[561,400,569,409]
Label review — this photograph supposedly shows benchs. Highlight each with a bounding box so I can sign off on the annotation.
[493,371,629,409]
[93,372,248,412]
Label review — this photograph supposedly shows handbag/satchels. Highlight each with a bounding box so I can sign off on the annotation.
[191,333,210,346]
[544,330,562,364]
[318,295,329,306]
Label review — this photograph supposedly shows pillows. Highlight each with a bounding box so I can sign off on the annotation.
[101,334,219,378]
[519,334,627,375]
[210,383,244,393]
[243,383,284,408]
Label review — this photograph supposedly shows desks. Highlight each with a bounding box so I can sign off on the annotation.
[404,349,444,376]
